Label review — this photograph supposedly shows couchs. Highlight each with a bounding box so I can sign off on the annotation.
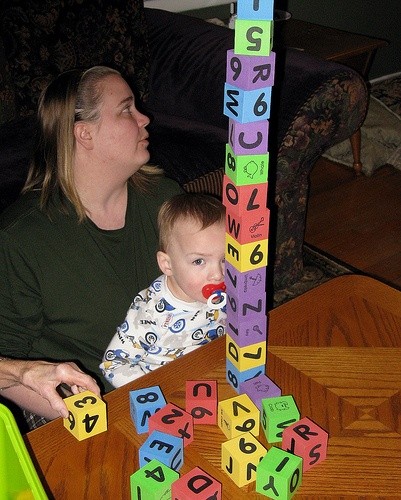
[0,1,369,287]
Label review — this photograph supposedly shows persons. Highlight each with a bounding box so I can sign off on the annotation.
[0,65,188,432]
[99,192,226,388]
[1,357,103,418]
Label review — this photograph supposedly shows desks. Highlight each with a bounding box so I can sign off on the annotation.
[201,15,390,176]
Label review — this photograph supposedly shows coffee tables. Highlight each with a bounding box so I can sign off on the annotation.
[16,274,401,499]
